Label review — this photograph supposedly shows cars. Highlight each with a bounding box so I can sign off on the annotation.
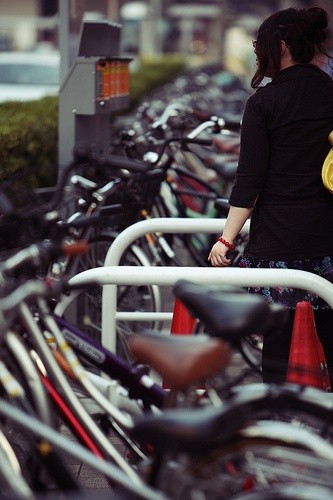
[1,51,61,102]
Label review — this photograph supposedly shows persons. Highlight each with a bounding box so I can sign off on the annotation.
[289,5,332,78]
[210,9,333,308]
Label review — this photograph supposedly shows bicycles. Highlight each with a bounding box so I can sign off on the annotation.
[1,62,332,499]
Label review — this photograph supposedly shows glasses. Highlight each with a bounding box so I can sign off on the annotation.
[252,39,257,47]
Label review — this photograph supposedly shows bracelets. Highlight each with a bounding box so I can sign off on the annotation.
[217,237,235,252]
